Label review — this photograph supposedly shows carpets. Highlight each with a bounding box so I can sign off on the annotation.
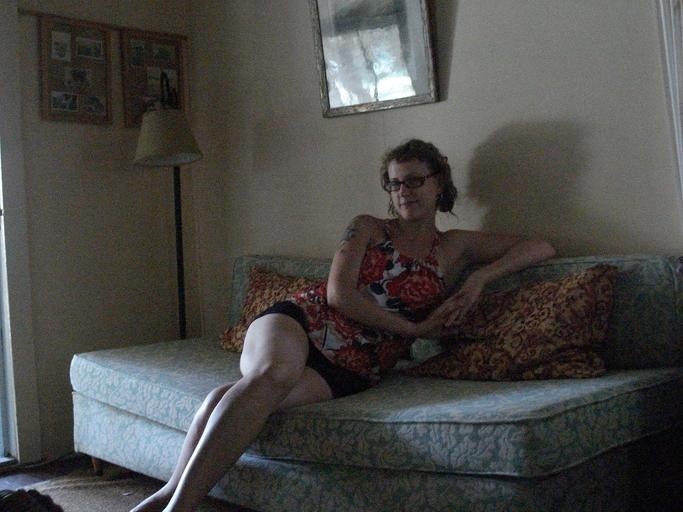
[11,466,260,512]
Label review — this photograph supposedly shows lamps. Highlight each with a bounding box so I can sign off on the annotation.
[132,108,204,340]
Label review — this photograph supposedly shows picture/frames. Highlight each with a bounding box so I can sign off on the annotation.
[38,15,113,124]
[120,27,189,129]
[307,0,440,118]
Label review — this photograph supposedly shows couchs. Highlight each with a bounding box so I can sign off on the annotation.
[69,253,683,512]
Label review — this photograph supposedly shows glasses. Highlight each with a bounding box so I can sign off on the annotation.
[383,171,437,193]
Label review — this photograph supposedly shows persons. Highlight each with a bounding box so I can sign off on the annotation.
[131,140,556,512]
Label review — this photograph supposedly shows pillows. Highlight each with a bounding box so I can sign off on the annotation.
[401,262,616,382]
[219,266,328,353]
